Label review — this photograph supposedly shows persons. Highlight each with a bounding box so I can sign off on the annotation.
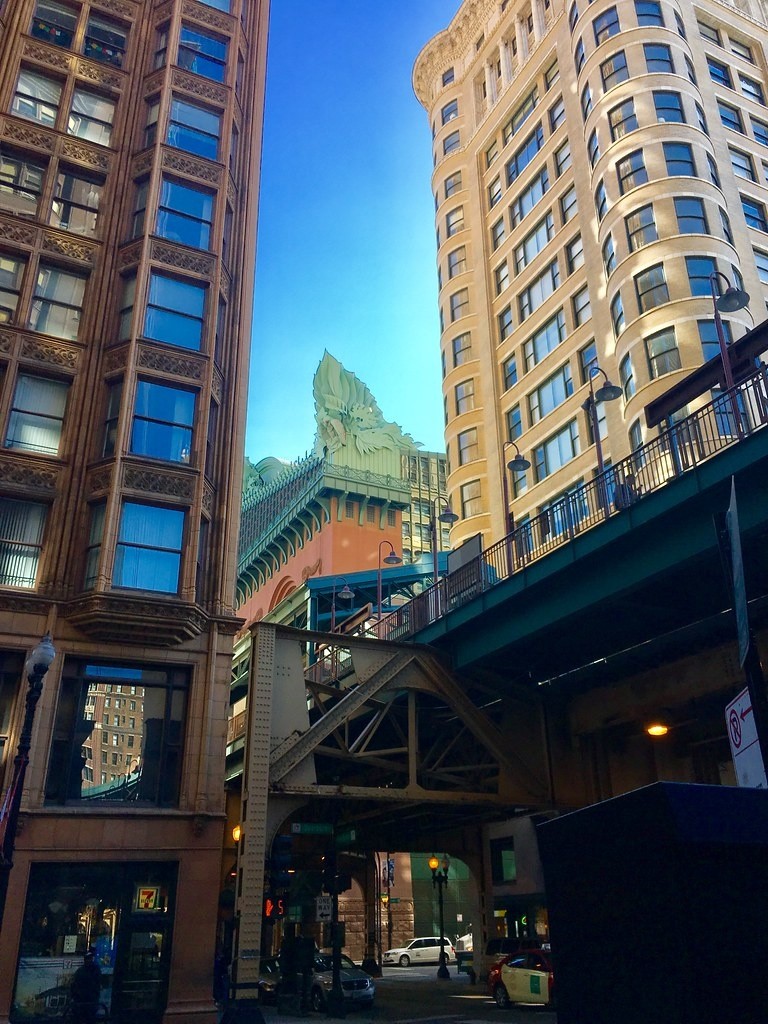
[70,953,101,1024]
[613,474,642,511]
[89,912,111,952]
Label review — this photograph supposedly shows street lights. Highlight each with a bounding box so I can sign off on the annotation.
[581,365,623,521]
[704,266,753,441]
[429,494,460,619]
[429,852,456,980]
[500,441,533,578]
[376,541,401,641]
[333,575,353,685]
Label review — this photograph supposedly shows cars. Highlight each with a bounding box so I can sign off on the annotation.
[296,953,376,1012]
[383,937,456,967]
[258,955,284,1002]
[487,948,559,1012]
